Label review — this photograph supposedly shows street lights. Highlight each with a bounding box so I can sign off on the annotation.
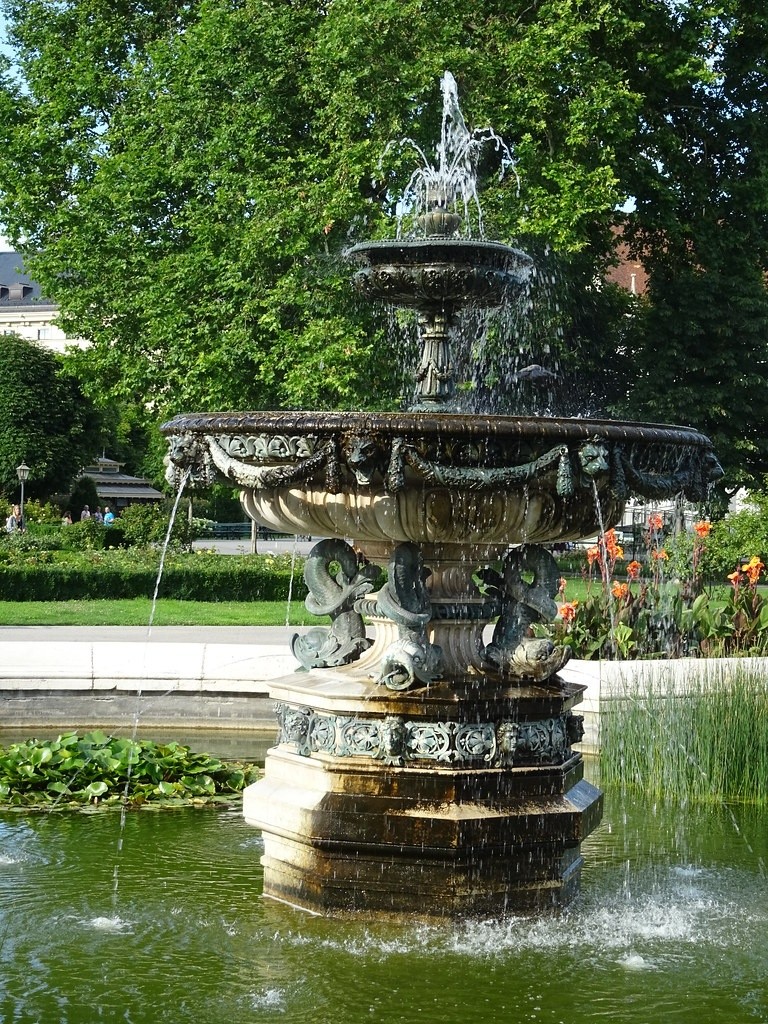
[17,461,31,529]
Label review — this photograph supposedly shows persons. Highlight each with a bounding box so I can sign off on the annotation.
[81,505,90,519]
[104,507,114,526]
[62,511,72,529]
[95,506,103,522]
[7,505,27,536]
